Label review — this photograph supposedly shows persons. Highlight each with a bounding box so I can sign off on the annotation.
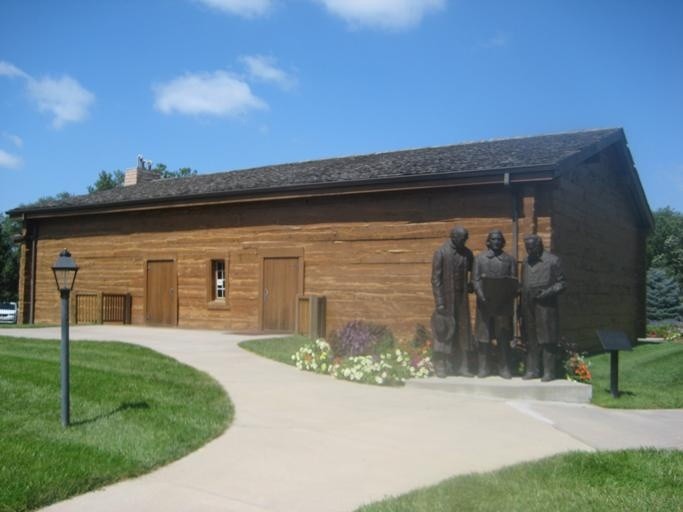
[518,235,568,382]
[431,226,474,377]
[473,229,518,378]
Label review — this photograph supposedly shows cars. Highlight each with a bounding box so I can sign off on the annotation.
[0,301,17,325]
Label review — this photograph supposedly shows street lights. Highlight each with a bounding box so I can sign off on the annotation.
[50,248,79,431]
